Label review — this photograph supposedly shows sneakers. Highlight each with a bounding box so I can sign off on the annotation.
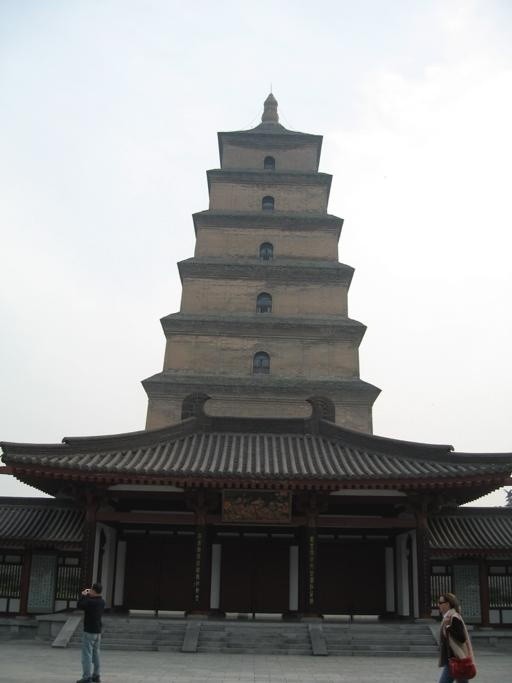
[77,674,100,683]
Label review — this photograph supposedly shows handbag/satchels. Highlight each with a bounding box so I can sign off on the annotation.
[449,657,476,679]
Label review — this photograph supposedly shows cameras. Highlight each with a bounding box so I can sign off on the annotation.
[86,589,91,593]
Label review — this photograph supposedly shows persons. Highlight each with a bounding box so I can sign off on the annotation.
[438,592,467,682]
[77,582,105,683]
